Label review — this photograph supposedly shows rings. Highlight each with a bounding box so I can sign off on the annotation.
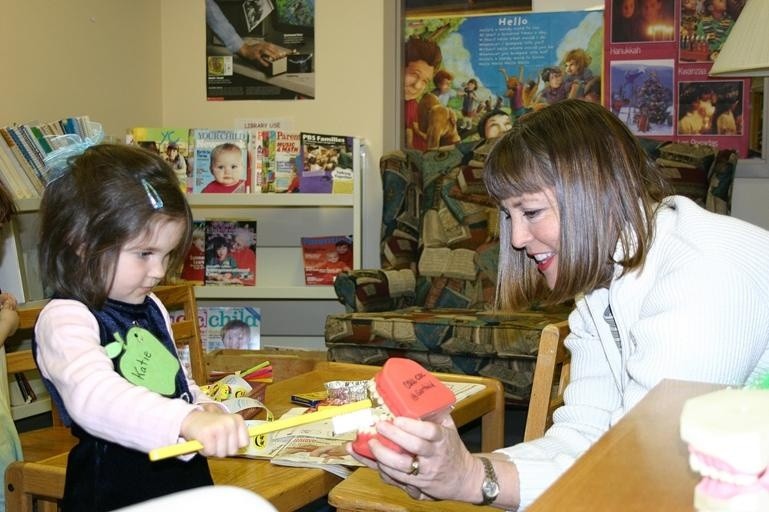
[408,455,421,476]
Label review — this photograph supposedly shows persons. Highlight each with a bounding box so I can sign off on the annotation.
[615,0,641,41]
[401,35,604,148]
[207,237,237,282]
[318,249,351,283]
[642,0,665,42]
[0,182,27,511]
[333,240,353,267]
[699,2,735,58]
[186,226,205,279]
[366,97,769,512]
[218,319,252,349]
[680,88,742,135]
[31,143,250,511]
[682,1,708,27]
[205,0,279,68]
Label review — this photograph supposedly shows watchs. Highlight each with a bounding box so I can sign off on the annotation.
[476,456,501,507]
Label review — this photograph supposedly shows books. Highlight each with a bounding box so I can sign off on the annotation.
[181,220,205,288]
[246,397,381,482]
[207,304,263,383]
[0,118,355,200]
[168,308,207,381]
[301,231,354,286]
[203,220,260,287]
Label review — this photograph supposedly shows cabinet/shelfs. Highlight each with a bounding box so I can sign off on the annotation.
[124,131,363,348]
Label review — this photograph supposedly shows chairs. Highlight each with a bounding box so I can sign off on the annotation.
[330,320,572,512]
[328,135,738,408]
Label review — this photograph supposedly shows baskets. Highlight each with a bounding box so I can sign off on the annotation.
[235,382,266,420]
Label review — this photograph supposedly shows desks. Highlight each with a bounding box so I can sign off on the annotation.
[6,349,503,509]
[518,375,744,512]
